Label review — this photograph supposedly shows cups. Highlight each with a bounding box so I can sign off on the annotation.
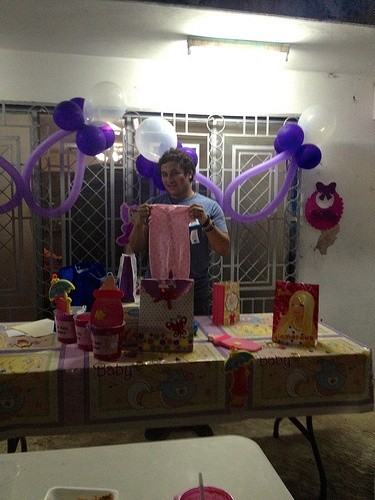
[173,486,235,500]
[53,308,76,344]
[74,312,93,352]
[88,320,126,360]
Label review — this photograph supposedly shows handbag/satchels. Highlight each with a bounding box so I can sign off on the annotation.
[213,281,239,326]
[138,278,194,353]
[271,280,320,347]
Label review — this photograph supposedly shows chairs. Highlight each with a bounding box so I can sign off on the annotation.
[59,262,106,312]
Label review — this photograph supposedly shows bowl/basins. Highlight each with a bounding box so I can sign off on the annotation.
[44,486,119,500]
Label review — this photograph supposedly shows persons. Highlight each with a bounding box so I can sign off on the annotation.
[128,147,230,442]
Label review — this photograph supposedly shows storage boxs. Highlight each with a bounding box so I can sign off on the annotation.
[213,282,240,326]
[44,486,119,500]
[139,278,194,352]
[122,303,140,345]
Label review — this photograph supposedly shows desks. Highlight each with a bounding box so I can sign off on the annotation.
[0,435,294,500]
[0,313,375,500]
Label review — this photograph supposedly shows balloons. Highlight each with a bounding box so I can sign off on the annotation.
[0,81,127,219]
[134,104,336,224]
